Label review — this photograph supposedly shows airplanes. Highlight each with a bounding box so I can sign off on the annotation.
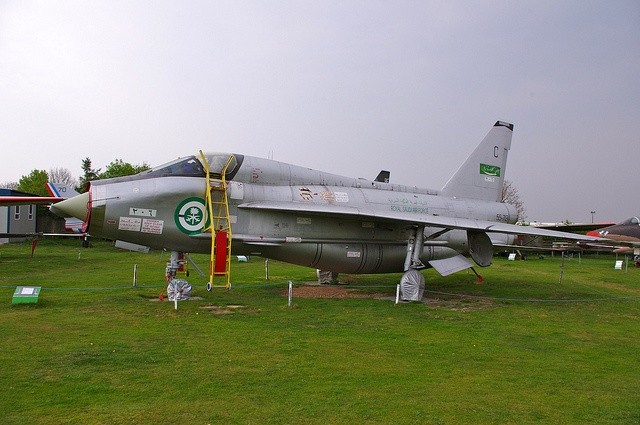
[48,119,614,304]
[586,215,640,268]
